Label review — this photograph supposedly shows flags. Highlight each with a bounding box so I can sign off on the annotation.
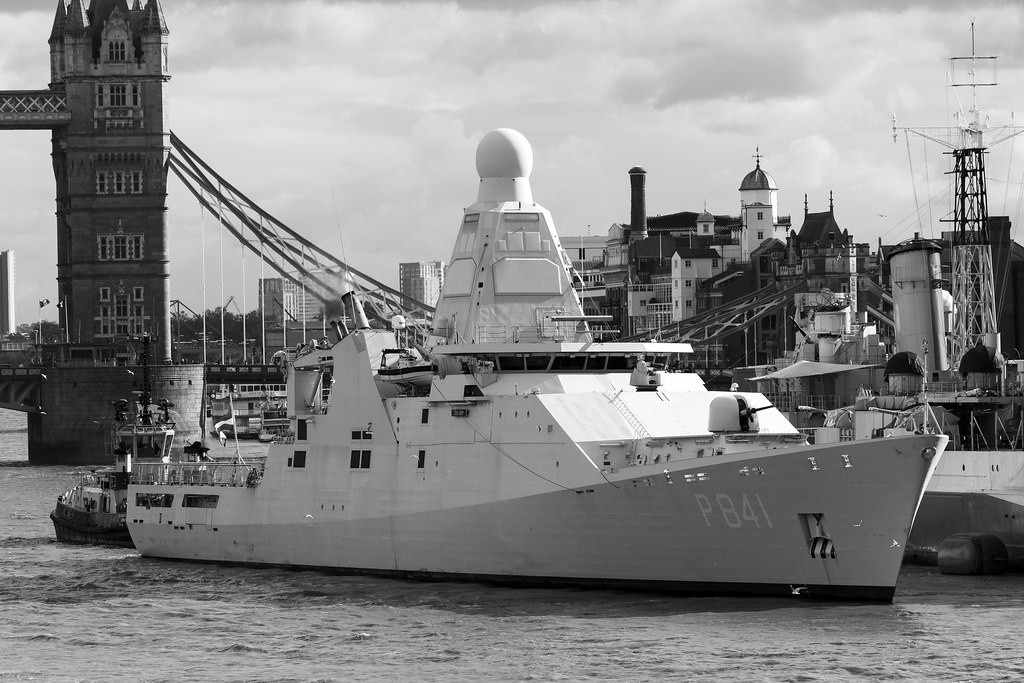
[40,299,50,308]
[212,395,233,446]
[56,300,65,308]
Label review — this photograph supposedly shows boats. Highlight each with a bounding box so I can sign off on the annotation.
[254,379,291,444]
[48,318,212,550]
[123,123,954,607]
[807,21,1023,578]
